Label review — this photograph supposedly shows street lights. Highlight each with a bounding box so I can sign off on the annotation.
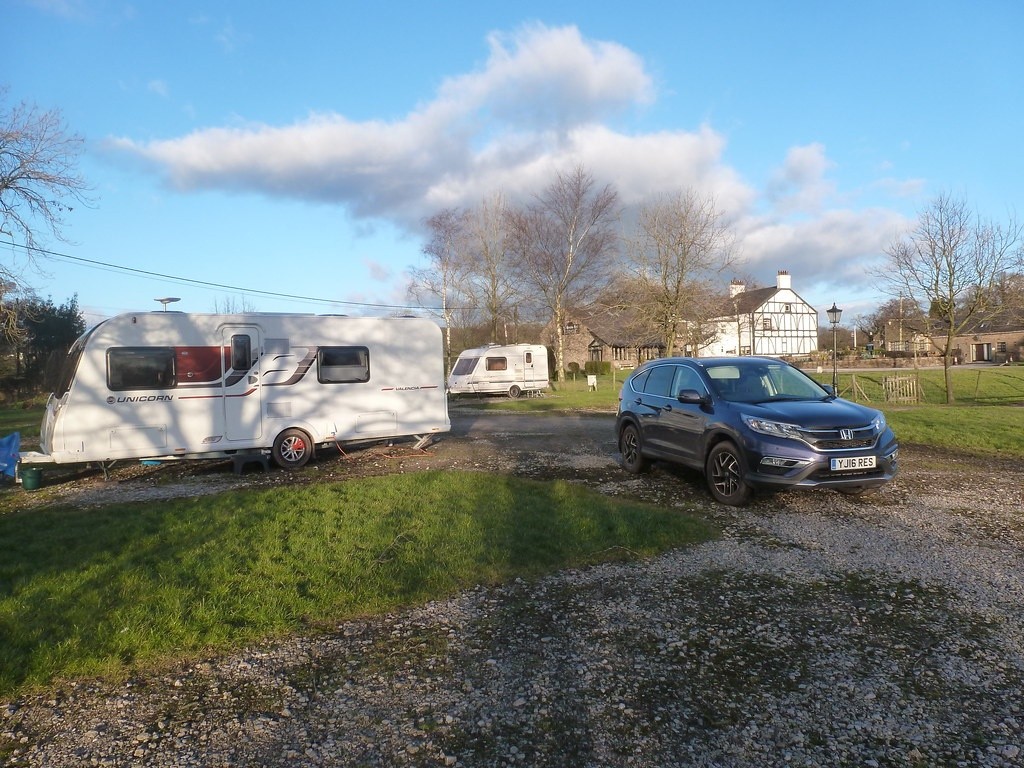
[825,301,845,394]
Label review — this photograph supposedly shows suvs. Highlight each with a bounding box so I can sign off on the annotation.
[615,355,900,505]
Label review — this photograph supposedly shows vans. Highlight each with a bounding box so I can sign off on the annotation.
[449,343,548,397]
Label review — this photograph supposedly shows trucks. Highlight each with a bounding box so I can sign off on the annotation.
[37,294,457,470]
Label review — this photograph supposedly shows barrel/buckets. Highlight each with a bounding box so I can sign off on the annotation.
[19,467,44,490]
